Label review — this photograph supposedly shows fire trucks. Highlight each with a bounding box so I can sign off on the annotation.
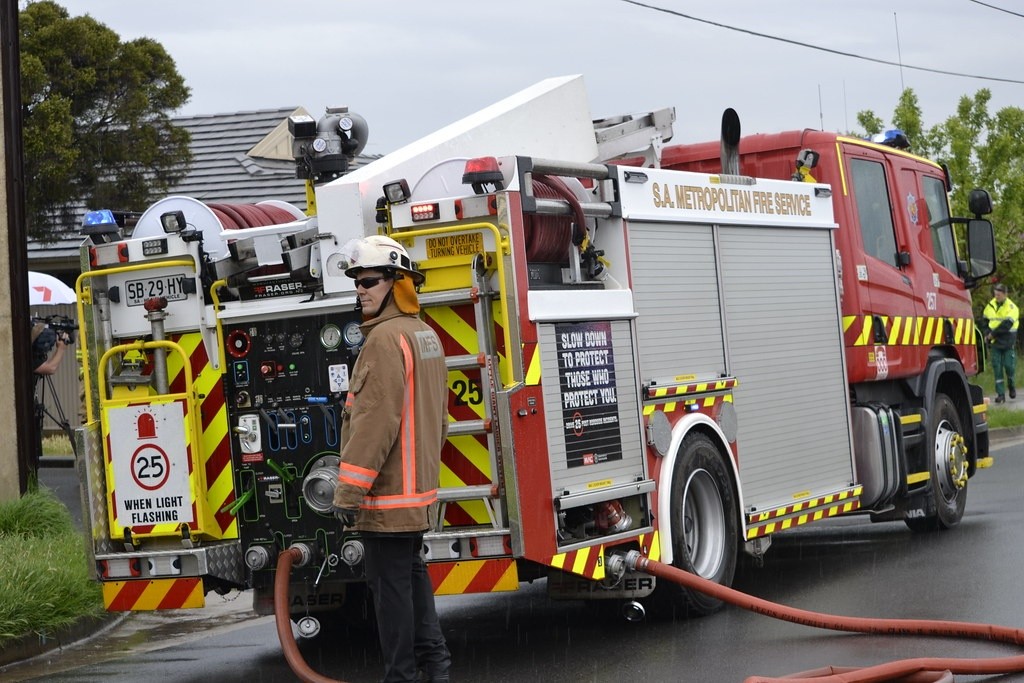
[76,74,998,645]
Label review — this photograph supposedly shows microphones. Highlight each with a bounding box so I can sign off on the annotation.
[54,323,79,330]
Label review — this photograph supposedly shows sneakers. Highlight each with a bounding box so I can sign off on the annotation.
[417,669,450,683]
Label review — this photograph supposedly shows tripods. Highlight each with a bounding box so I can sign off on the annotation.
[39,373,79,461]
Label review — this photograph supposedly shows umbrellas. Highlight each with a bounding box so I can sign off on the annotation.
[27,271,85,306]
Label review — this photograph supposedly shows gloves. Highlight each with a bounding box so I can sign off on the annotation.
[334,507,355,528]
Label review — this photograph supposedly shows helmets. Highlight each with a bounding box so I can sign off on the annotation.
[345,235,425,286]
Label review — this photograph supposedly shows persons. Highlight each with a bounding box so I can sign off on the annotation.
[326,235,452,683]
[24,322,69,402]
[982,283,1020,404]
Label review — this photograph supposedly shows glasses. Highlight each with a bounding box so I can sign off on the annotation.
[994,287,1005,291]
[354,277,385,289]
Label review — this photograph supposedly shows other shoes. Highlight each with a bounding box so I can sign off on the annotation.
[1009,388,1016,399]
[995,393,1005,403]
